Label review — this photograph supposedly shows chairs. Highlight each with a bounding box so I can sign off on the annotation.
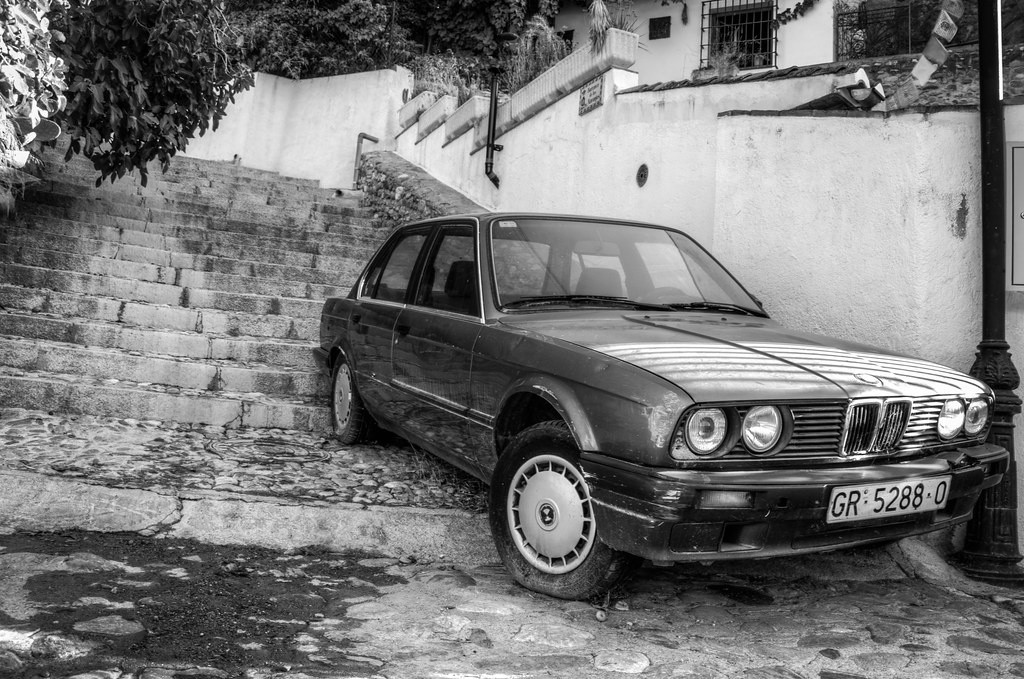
[575,267,624,298]
[444,260,475,298]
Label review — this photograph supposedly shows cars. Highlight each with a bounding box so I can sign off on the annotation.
[312,211,1009,600]
